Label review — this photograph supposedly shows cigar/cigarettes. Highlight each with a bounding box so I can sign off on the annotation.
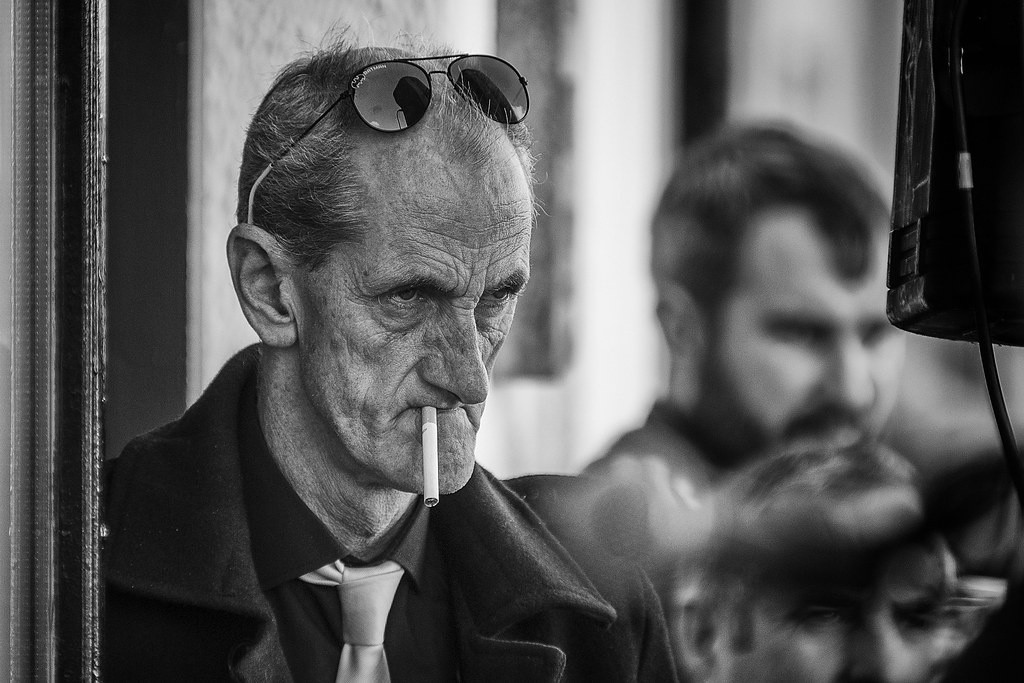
[418,406,440,506]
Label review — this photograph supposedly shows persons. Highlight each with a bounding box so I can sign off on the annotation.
[576,115,1024,683]
[53,15,679,683]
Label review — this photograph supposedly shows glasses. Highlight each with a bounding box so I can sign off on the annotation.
[248,54,530,226]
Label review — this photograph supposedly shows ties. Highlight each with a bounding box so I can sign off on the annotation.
[300,558,405,683]
